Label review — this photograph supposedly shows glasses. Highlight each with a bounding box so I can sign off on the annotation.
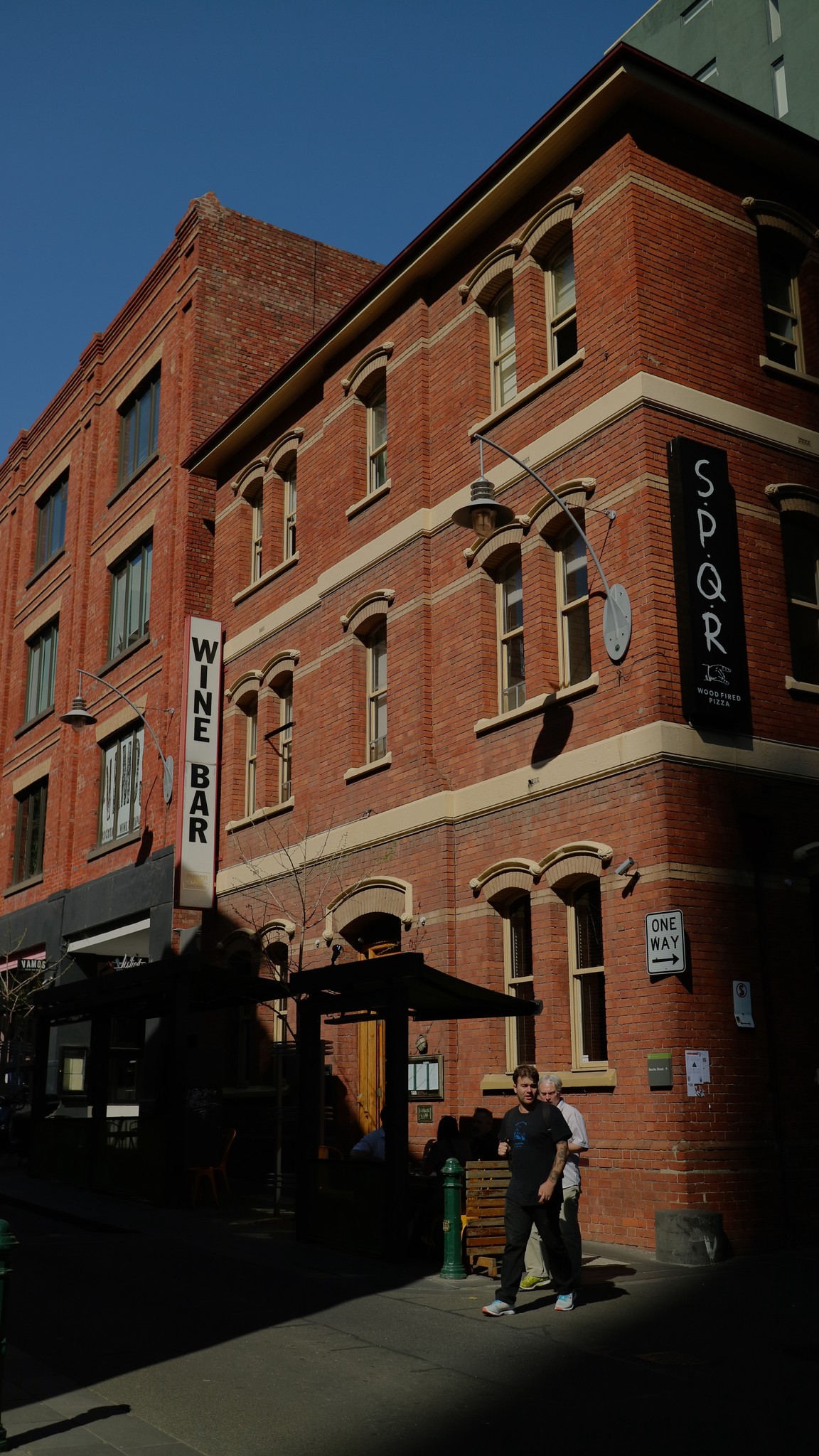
[441,1116,456,1119]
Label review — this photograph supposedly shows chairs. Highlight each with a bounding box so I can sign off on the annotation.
[186,1127,237,1206]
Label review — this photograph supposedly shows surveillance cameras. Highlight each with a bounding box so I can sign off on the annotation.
[614,857,635,876]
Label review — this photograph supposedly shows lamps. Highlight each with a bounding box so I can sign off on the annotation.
[58,670,173,810]
[446,434,632,663]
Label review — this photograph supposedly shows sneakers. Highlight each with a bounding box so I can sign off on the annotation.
[555,1289,577,1311]
[482,1300,516,1316]
[519,1276,551,1290]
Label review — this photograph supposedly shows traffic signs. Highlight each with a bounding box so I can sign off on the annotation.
[644,909,688,974]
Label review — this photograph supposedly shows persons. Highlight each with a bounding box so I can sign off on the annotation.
[349,1106,386,1160]
[422,1116,472,1208]
[482,1065,577,1316]
[469,1108,504,1161]
[517,1076,590,1290]
